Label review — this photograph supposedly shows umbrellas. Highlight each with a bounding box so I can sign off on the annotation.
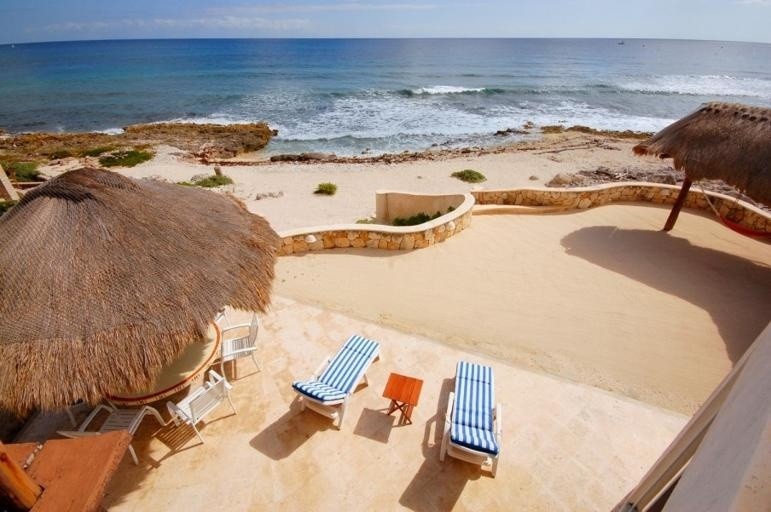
[0,168,286,420]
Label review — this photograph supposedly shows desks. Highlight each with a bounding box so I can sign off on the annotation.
[382,372,424,424]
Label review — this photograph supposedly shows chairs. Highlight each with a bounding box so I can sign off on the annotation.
[441,361,502,477]
[57,306,260,462]
[290,333,382,429]
